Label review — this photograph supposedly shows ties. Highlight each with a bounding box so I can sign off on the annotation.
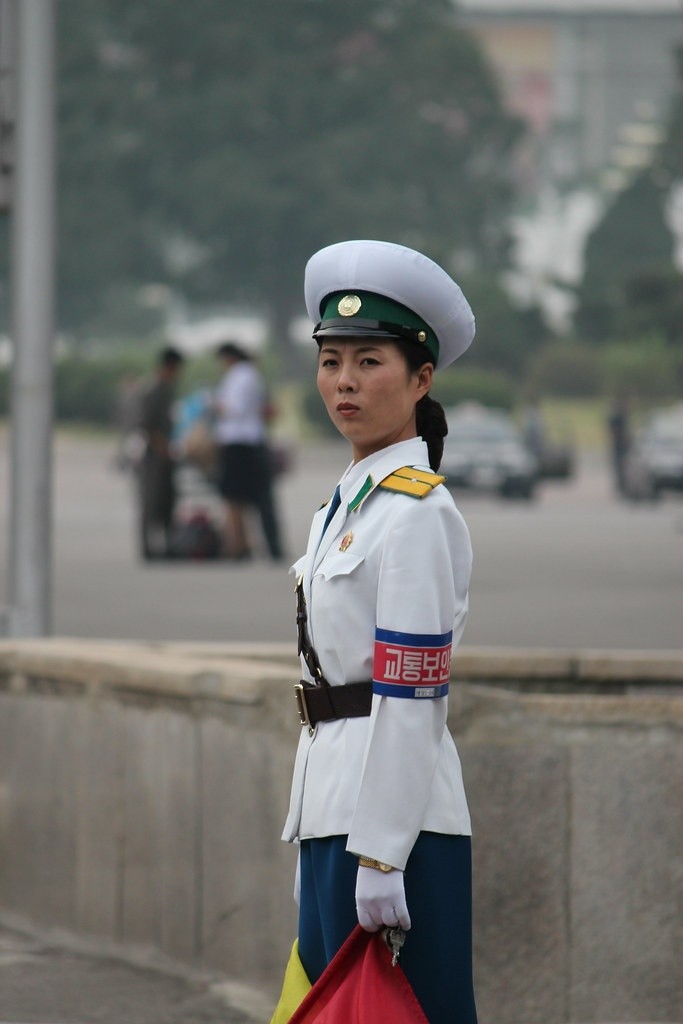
[316,485,347,571]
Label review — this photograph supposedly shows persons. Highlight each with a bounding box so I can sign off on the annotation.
[135,345,188,565]
[276,238,480,1022]
[203,343,288,565]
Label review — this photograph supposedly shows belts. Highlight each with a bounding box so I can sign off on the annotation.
[287,675,379,731]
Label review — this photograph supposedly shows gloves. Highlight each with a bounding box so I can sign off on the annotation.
[353,855,412,937]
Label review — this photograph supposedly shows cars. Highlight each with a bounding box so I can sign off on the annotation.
[438,399,541,499]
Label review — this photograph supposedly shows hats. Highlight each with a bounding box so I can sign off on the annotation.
[299,239,479,373]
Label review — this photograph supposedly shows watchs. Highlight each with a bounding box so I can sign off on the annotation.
[358,858,398,875]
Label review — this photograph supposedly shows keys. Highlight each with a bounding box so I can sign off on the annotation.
[385,925,406,967]
